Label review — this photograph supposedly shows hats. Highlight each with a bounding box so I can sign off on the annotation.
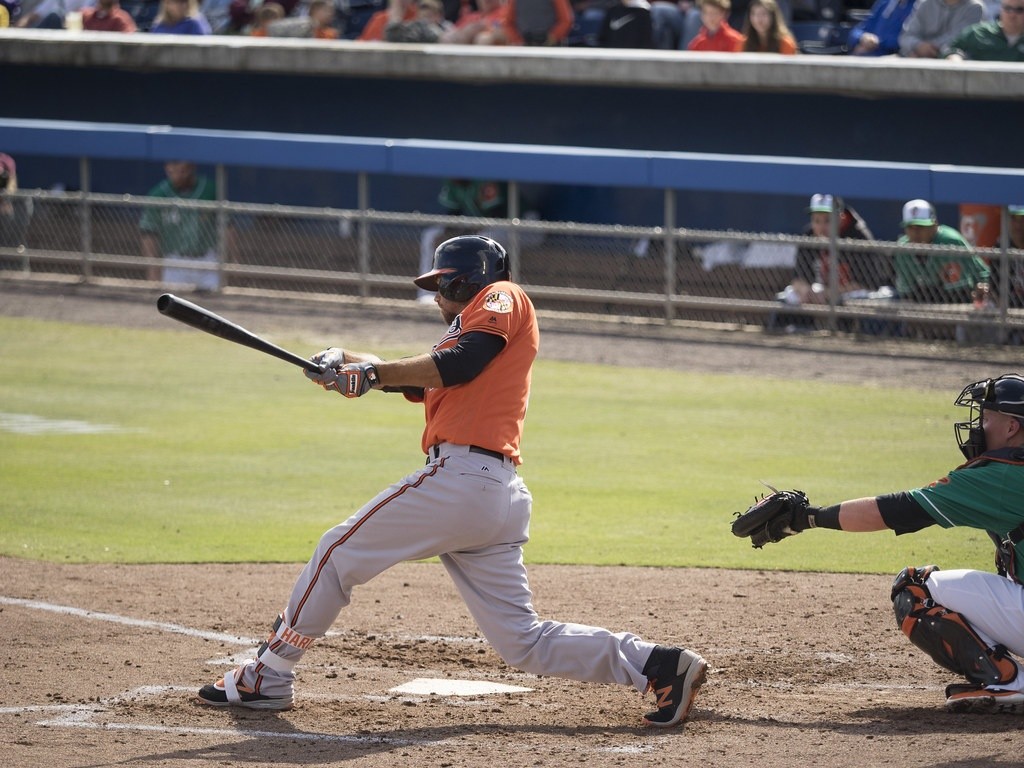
[807,192,837,212]
[899,199,938,228]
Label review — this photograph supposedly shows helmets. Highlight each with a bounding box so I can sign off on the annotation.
[970,373,1024,417]
[412,234,509,301]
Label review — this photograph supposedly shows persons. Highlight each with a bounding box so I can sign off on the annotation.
[0,153,36,273]
[765,192,900,335]
[892,197,992,342]
[415,176,550,304]
[731,373,1024,706]
[136,162,241,287]
[956,159,1024,350]
[0,0,1024,63]
[198,234,711,726]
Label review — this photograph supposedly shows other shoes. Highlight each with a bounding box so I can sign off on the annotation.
[945,688,1024,715]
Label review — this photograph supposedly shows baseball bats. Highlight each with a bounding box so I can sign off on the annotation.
[156,292,322,372]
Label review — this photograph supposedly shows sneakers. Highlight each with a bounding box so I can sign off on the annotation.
[644,646,710,727]
[198,669,294,710]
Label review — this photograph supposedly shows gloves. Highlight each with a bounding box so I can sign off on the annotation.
[304,347,345,384]
[331,362,380,399]
